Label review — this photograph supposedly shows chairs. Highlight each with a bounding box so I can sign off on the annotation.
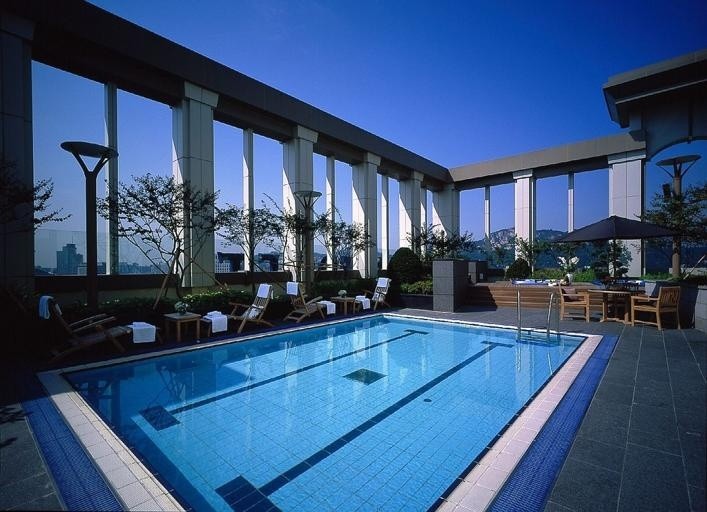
[353,277,393,312]
[37,294,159,358]
[556,278,592,324]
[282,281,336,323]
[200,283,275,336]
[630,283,682,331]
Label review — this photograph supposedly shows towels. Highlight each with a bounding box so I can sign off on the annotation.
[201,309,229,333]
[286,281,299,297]
[355,295,371,309]
[256,283,274,303]
[376,277,389,288]
[127,319,156,345]
[316,300,336,315]
[38,294,61,317]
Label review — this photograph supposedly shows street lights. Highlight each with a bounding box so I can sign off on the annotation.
[656,155,701,278]
[293,191,322,299]
[61,142,119,306]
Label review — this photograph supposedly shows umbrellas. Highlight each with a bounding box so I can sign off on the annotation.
[557,215,682,278]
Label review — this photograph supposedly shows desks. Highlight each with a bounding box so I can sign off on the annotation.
[588,286,645,325]
[161,311,201,342]
[331,297,356,316]
